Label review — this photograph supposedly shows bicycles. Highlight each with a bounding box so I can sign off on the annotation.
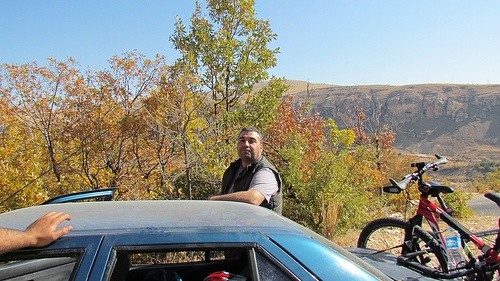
[356,146,499,280]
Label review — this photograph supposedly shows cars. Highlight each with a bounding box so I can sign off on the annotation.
[1,188,445,280]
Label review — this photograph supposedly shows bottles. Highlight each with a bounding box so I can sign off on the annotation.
[446,225,467,279]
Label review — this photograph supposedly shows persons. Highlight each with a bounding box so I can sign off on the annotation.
[207,127,282,216]
[0,210,72,256]
